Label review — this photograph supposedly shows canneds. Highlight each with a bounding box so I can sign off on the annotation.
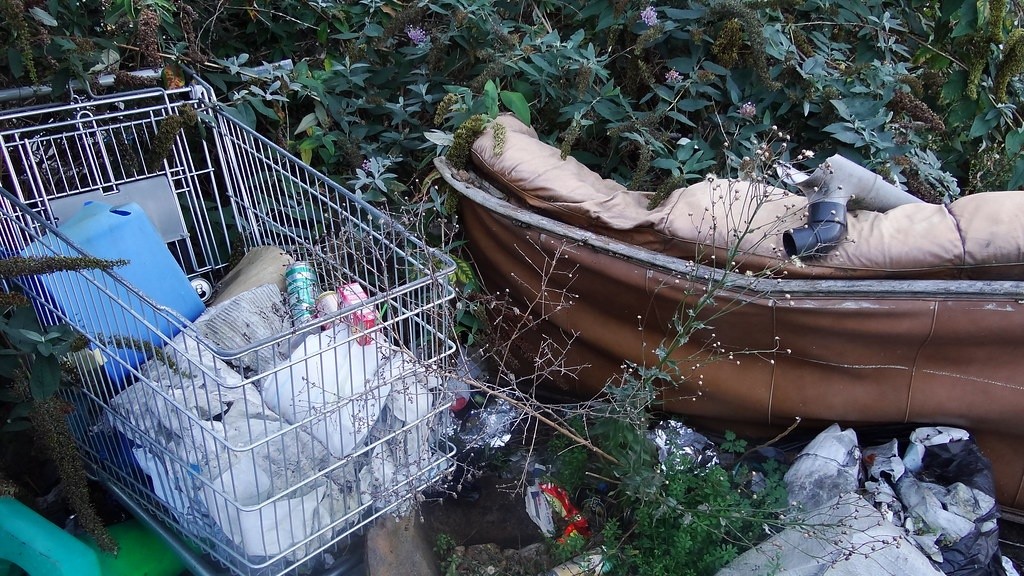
[285,262,321,328]
[315,291,344,329]
[188,279,211,302]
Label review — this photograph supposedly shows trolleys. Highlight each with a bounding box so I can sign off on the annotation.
[0,64,458,576]
